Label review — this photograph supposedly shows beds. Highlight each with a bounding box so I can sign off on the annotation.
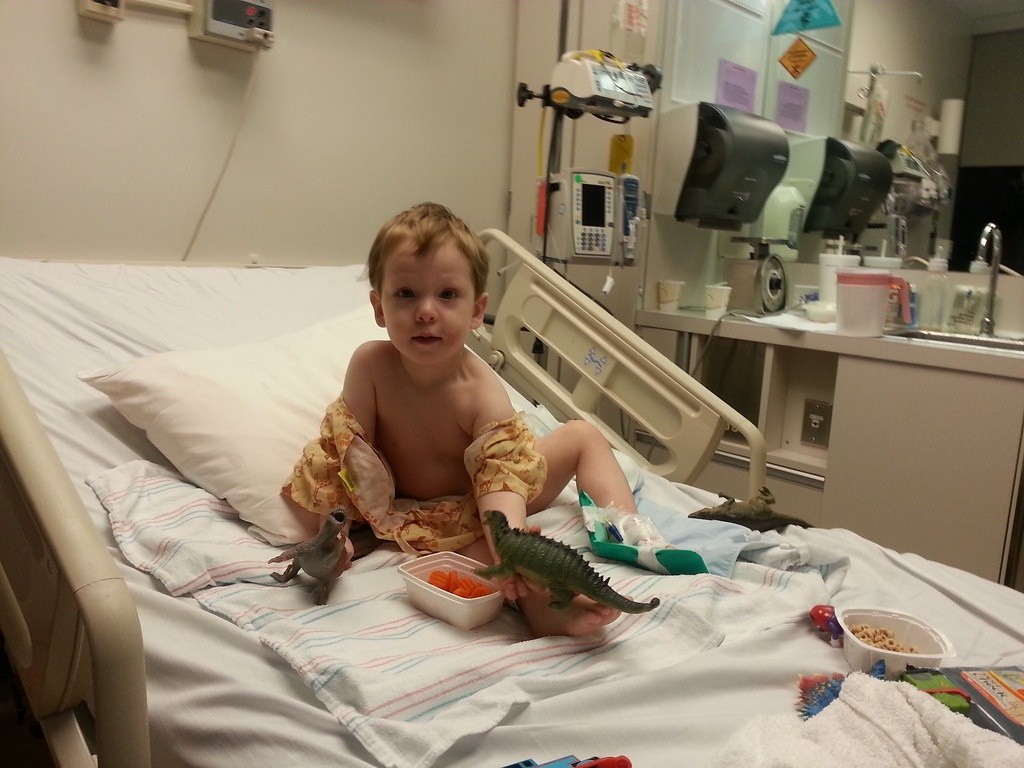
[0,228,1024,768]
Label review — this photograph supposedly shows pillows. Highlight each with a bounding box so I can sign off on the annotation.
[77,303,391,547]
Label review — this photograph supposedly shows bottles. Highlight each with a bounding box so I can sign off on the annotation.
[919,257,950,329]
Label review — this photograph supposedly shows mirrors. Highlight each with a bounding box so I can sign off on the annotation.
[636,1,1024,334]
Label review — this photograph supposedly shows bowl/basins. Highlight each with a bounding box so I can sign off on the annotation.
[835,605,957,681]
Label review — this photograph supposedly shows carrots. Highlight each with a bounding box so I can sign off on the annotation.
[425,569,493,598]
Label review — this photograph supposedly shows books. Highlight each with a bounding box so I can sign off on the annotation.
[941,666,1024,746]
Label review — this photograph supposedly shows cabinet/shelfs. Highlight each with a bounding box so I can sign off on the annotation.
[819,336,1023,590]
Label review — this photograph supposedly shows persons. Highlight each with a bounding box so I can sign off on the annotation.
[281,202,710,638]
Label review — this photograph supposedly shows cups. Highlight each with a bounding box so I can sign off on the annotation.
[703,285,732,320]
[864,255,902,270]
[819,254,859,305]
[659,281,685,313]
[793,285,819,309]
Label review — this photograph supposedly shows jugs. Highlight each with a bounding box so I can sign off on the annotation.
[836,268,912,337]
